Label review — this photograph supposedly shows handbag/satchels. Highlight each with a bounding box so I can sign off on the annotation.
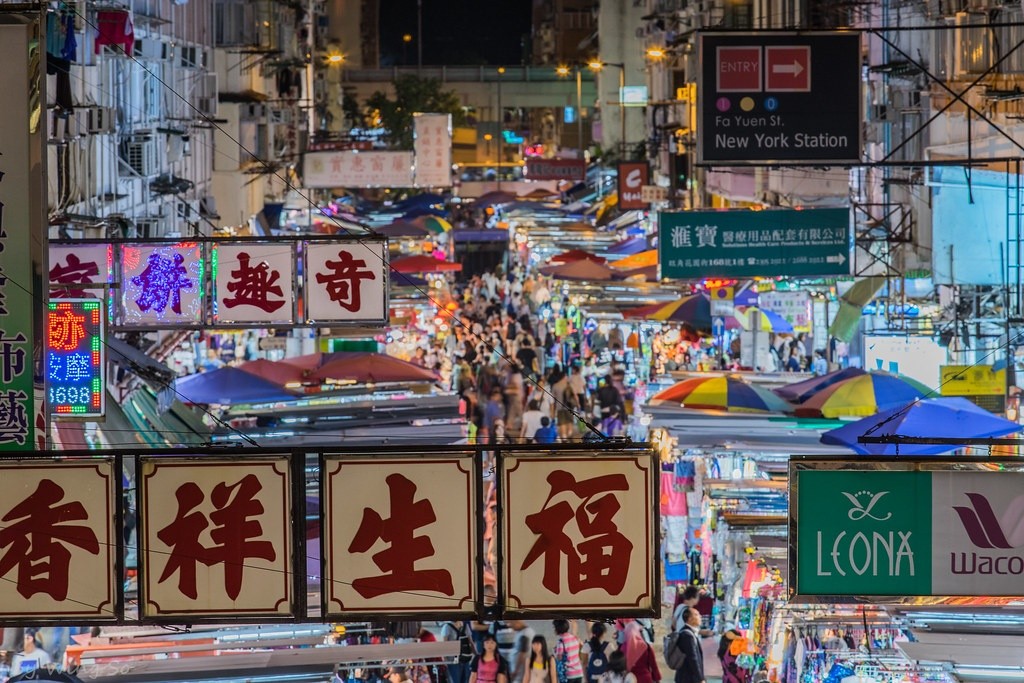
[556,639,568,682]
[564,382,576,410]
[636,620,655,642]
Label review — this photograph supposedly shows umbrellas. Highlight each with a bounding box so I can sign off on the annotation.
[295,191,457,237]
[541,245,791,332]
[169,350,441,405]
[651,365,1024,458]
[474,185,570,217]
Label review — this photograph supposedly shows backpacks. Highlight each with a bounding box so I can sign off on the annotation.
[663,629,698,670]
[491,621,516,649]
[587,641,609,680]
[448,622,473,663]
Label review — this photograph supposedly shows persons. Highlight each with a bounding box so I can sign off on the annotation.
[163,327,256,372]
[453,254,627,444]
[338,584,751,683]
[652,328,827,374]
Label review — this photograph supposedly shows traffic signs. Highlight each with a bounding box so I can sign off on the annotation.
[696,32,862,167]
